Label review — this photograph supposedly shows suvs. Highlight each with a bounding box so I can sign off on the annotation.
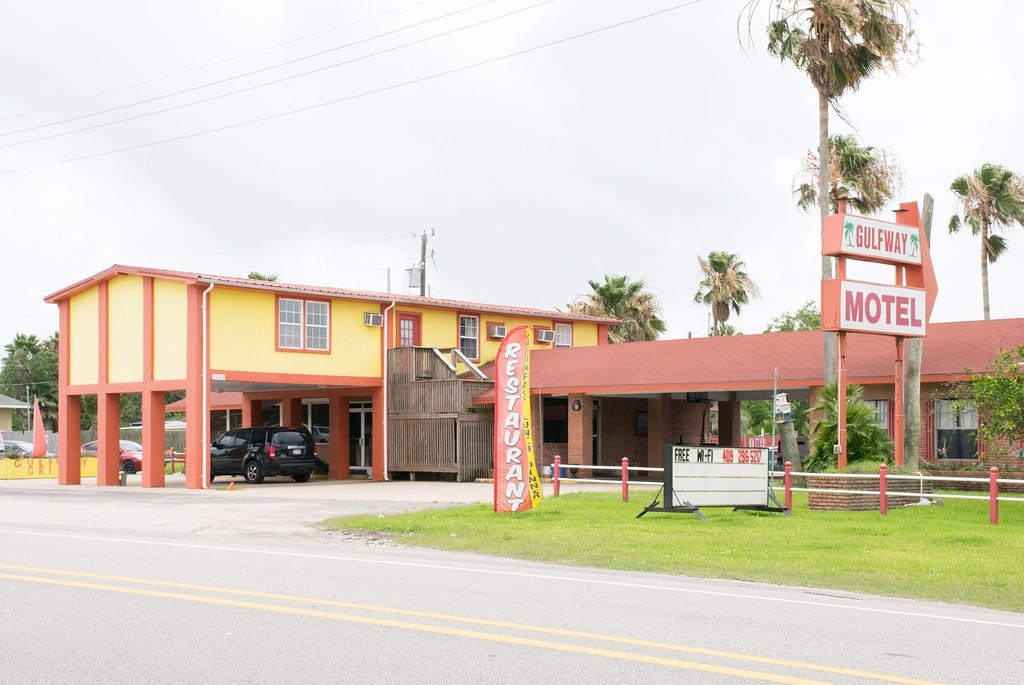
[210,427,317,484]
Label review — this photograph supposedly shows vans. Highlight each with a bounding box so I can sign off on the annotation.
[776,436,809,463]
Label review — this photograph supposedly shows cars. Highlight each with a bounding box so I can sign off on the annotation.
[1,440,58,458]
[80,439,143,475]
[313,425,329,442]
[768,448,778,466]
